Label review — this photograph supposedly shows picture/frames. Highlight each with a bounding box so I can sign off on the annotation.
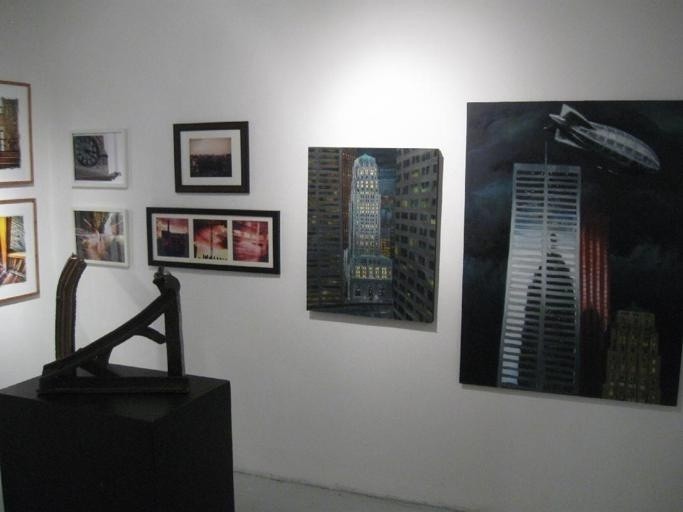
[69,208,129,269]
[0,198,40,306]
[66,129,128,190]
[146,206,281,273]
[0,80,34,188]
[173,120,250,194]
